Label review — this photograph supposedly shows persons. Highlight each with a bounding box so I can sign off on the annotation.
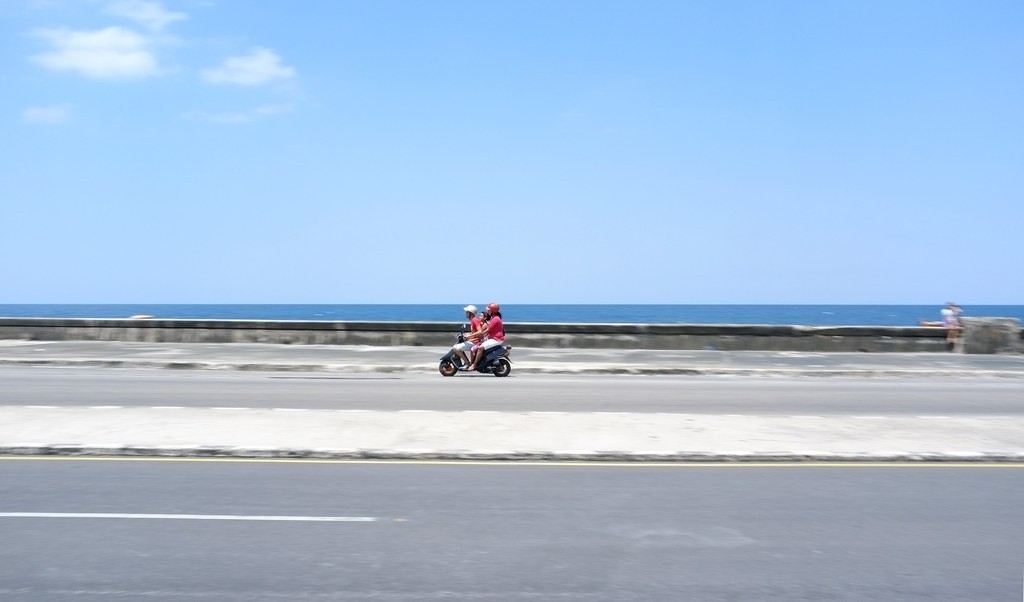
[919,303,963,343]
[452,303,504,371]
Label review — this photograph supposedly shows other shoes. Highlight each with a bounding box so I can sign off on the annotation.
[468,364,477,370]
[459,363,470,370]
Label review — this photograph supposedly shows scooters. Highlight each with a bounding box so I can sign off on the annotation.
[438,324,514,377]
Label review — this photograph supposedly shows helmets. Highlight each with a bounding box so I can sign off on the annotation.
[463,305,478,317]
[485,303,499,313]
